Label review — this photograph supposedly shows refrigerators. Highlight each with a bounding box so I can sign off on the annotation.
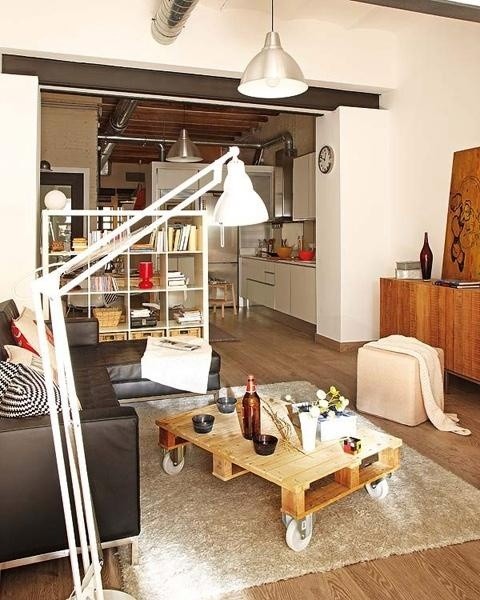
[199,191,239,307]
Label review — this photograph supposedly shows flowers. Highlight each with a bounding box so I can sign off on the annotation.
[284,385,350,417]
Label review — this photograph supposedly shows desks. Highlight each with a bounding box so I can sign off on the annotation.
[380,278,480,394]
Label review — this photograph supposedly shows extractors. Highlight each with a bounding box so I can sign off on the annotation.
[241,215,304,225]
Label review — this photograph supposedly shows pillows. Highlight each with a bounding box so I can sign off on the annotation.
[0,363,62,419]
[0,360,19,398]
[3,344,58,384]
[10,306,53,357]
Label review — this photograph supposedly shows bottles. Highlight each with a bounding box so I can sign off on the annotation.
[241,374,261,441]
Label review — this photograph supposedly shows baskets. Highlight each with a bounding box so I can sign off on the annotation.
[93,307,122,327]
[131,331,164,339]
[170,327,201,337]
[99,332,127,343]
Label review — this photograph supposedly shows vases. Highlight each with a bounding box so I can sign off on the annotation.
[292,401,358,442]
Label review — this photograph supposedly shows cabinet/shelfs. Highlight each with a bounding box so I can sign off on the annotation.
[41,208,210,345]
[240,256,317,326]
[292,152,316,221]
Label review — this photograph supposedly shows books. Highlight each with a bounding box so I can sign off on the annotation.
[130,308,152,318]
[291,398,358,444]
[89,222,202,253]
[91,273,119,293]
[168,271,202,328]
[434,279,480,286]
[432,281,480,288]
[153,339,200,351]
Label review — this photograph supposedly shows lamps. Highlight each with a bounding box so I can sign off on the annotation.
[14,145,270,600]
[237,0,309,99]
[165,103,204,163]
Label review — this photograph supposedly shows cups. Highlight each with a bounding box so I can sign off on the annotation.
[191,414,214,433]
[253,434,277,455]
[216,397,237,413]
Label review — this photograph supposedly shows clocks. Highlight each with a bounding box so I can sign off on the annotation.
[318,145,335,175]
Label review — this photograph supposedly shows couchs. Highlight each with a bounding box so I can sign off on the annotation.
[0,299,221,571]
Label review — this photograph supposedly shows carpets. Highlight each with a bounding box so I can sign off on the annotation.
[210,322,240,343]
[115,380,480,600]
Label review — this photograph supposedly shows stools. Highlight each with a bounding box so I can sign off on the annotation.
[356,346,445,427]
[209,282,237,318]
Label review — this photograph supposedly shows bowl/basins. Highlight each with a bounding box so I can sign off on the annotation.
[276,247,292,257]
[297,250,315,260]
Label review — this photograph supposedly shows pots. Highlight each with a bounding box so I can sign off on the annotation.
[261,251,279,258]
